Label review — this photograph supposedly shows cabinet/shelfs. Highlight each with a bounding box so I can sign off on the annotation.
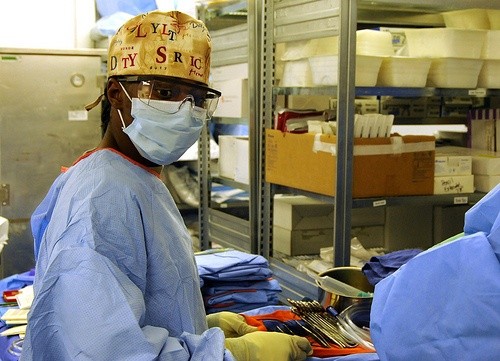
[195,0,259,255]
[257,0,500,267]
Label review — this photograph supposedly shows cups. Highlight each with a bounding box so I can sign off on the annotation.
[315,266,374,312]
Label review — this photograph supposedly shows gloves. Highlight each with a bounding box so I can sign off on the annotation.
[206,311,259,338]
[224,330,312,361]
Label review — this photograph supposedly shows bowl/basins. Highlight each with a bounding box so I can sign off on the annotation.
[279,8,500,90]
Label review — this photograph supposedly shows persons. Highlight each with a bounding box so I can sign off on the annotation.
[19,10,313,361]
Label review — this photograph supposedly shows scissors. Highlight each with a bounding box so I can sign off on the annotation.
[288,298,357,349]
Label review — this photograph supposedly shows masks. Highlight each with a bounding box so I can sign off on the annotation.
[118,81,207,166]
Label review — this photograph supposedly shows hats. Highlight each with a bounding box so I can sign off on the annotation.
[107,10,211,86]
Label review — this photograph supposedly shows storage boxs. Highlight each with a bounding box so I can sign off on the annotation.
[264,127,500,257]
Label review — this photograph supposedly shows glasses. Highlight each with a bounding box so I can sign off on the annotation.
[115,75,221,120]
